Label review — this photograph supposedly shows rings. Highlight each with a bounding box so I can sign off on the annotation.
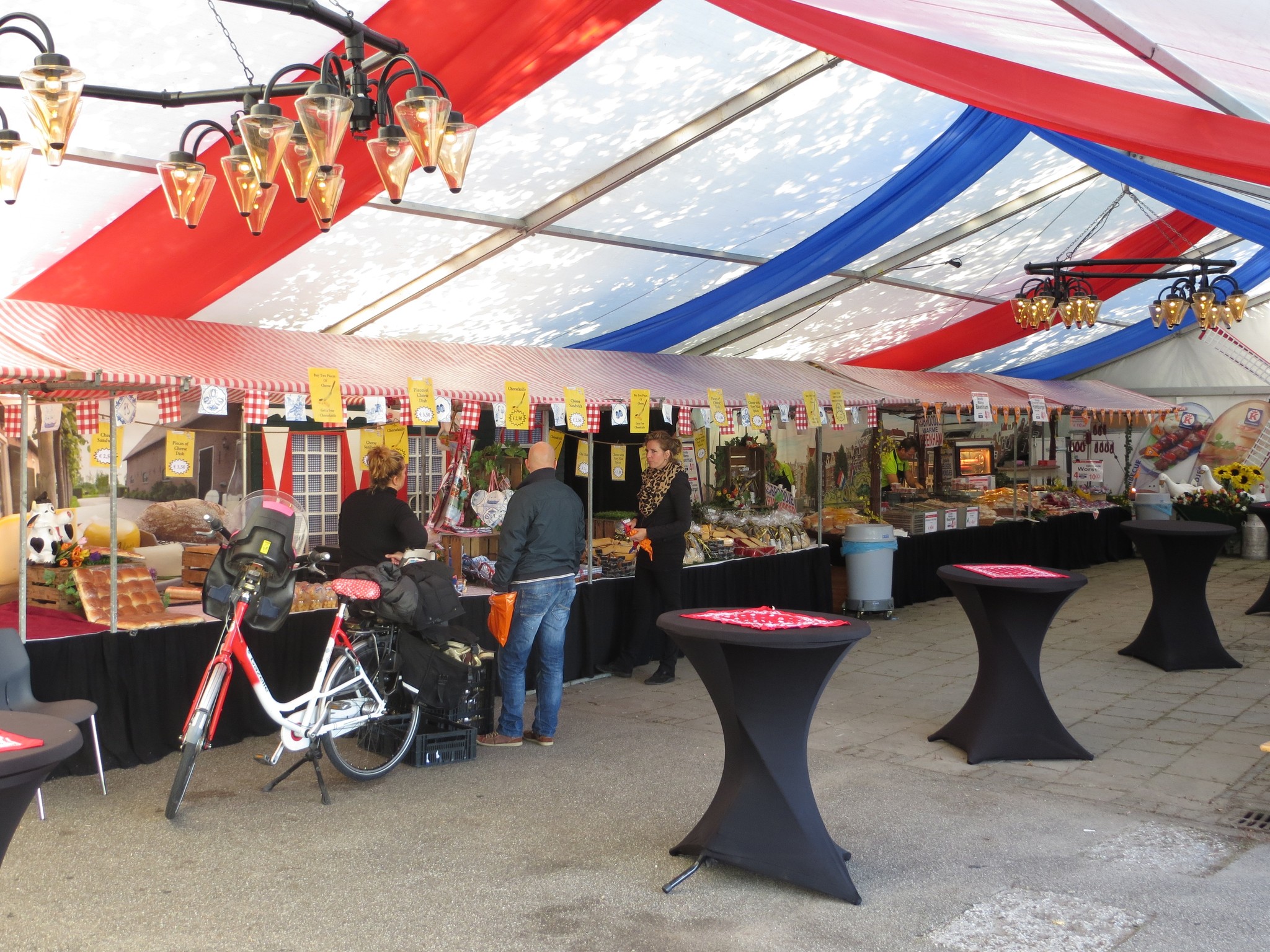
[635,538,638,541]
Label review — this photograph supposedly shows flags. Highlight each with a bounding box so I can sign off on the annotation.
[850,465,856,485]
[836,470,846,488]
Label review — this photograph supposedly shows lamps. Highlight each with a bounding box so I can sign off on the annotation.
[1009,183,1246,335]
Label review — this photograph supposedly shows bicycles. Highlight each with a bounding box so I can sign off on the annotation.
[166,489,421,820]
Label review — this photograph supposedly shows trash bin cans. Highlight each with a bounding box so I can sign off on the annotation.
[1129,493,1174,521]
[840,524,897,621]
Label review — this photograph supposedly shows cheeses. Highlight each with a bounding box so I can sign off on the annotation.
[699,522,733,548]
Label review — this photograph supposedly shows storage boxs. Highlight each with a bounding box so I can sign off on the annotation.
[356,712,475,766]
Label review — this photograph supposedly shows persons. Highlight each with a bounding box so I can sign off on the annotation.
[594,430,693,685]
[881,437,925,502]
[338,446,429,738]
[763,440,795,495]
[476,441,586,747]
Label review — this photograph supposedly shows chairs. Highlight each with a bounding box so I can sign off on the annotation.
[0,627,105,823]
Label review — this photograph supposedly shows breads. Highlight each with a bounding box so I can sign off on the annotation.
[802,506,867,534]
[73,564,203,631]
[901,486,1041,519]
[290,580,338,612]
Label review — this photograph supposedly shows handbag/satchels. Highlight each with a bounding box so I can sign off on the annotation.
[396,624,482,711]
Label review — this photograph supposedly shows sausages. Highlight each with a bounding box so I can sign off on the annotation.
[714,525,776,558]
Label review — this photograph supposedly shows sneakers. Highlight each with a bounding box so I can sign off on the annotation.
[523,730,554,747]
[644,666,675,685]
[476,729,523,746]
[595,655,634,678]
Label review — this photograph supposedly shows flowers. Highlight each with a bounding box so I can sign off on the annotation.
[1173,462,1266,512]
